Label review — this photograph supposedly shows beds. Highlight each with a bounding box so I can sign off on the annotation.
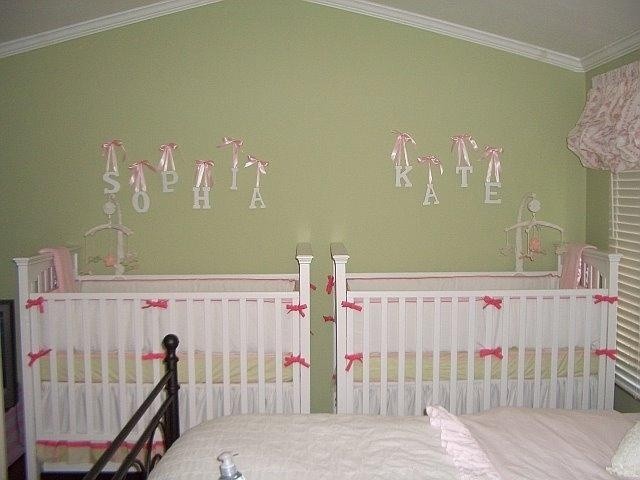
[79,332,640,478]
[327,239,622,414]
[9,239,315,480]
